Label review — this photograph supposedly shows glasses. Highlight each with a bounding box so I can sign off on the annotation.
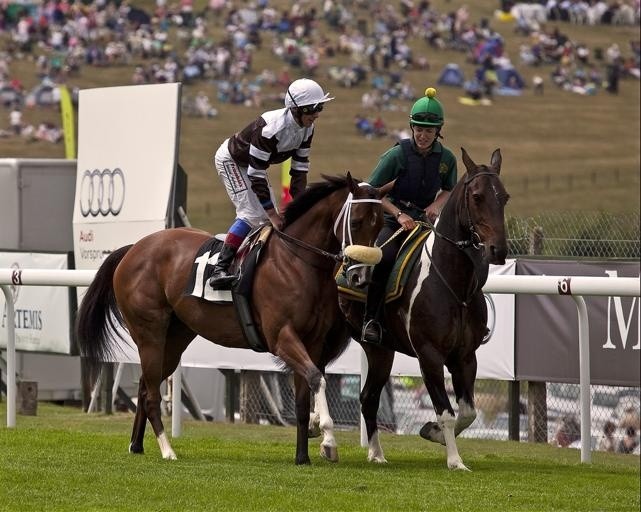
[304,105,325,115]
[408,113,442,123]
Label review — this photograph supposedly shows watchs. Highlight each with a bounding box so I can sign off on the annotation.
[395,210,405,221]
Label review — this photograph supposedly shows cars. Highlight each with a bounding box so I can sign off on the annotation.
[337,375,640,455]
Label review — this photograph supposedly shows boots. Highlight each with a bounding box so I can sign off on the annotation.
[208,243,239,288]
[360,283,384,344]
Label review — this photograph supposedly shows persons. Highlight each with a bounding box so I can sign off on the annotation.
[0,0,639,146]
[208,76,335,287]
[359,86,457,344]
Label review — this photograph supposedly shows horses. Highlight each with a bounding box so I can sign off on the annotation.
[72,168,398,468]
[306,145,511,474]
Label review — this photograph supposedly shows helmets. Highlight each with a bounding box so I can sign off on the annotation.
[410,88,445,128]
[284,77,335,109]
